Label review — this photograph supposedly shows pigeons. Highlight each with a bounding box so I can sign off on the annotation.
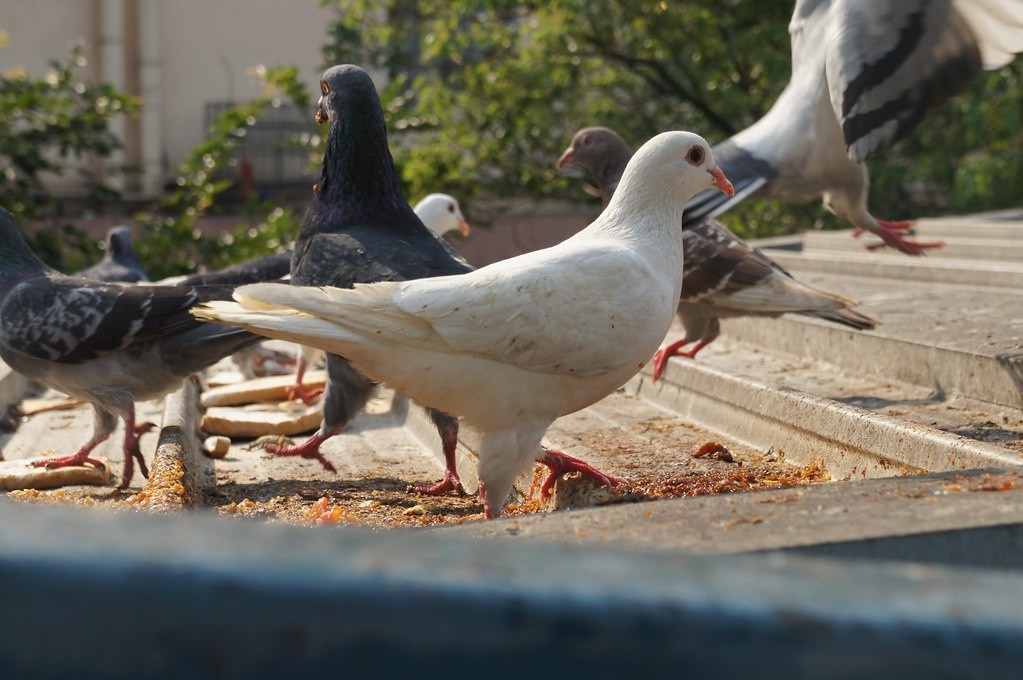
[0,0,1023,522]
